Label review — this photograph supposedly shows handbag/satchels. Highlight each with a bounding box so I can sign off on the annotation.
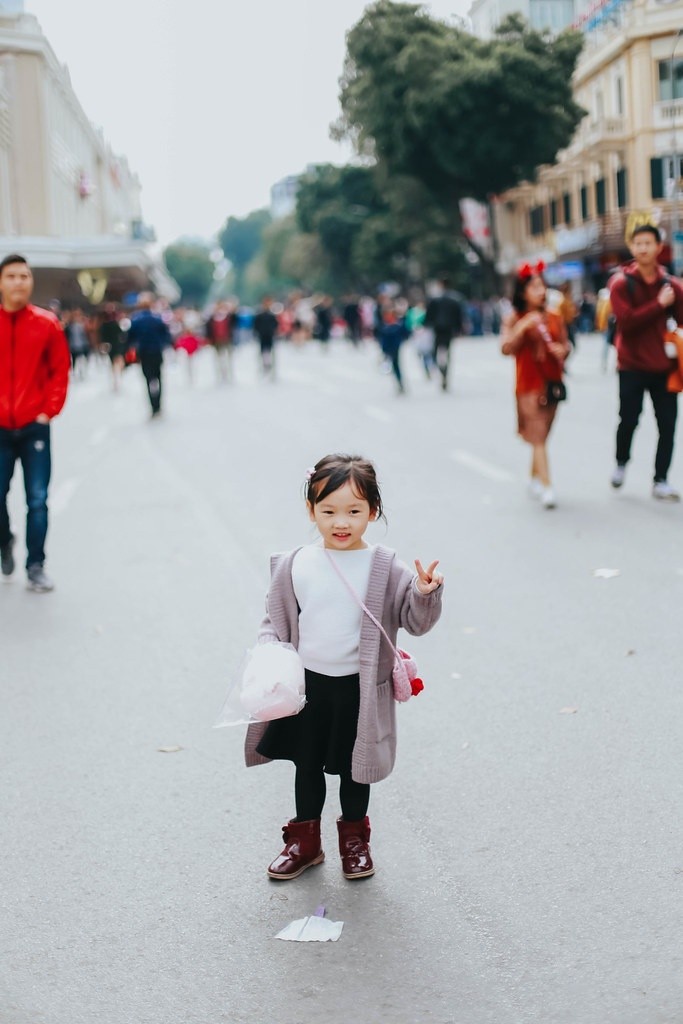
[391,649,424,702]
[544,379,567,402]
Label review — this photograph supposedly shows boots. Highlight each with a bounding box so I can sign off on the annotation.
[337,814,375,879]
[267,816,325,880]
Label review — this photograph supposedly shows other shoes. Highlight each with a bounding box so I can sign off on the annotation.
[1,536,15,575]
[653,483,678,501]
[613,465,625,486]
[541,488,555,507]
[27,565,53,592]
